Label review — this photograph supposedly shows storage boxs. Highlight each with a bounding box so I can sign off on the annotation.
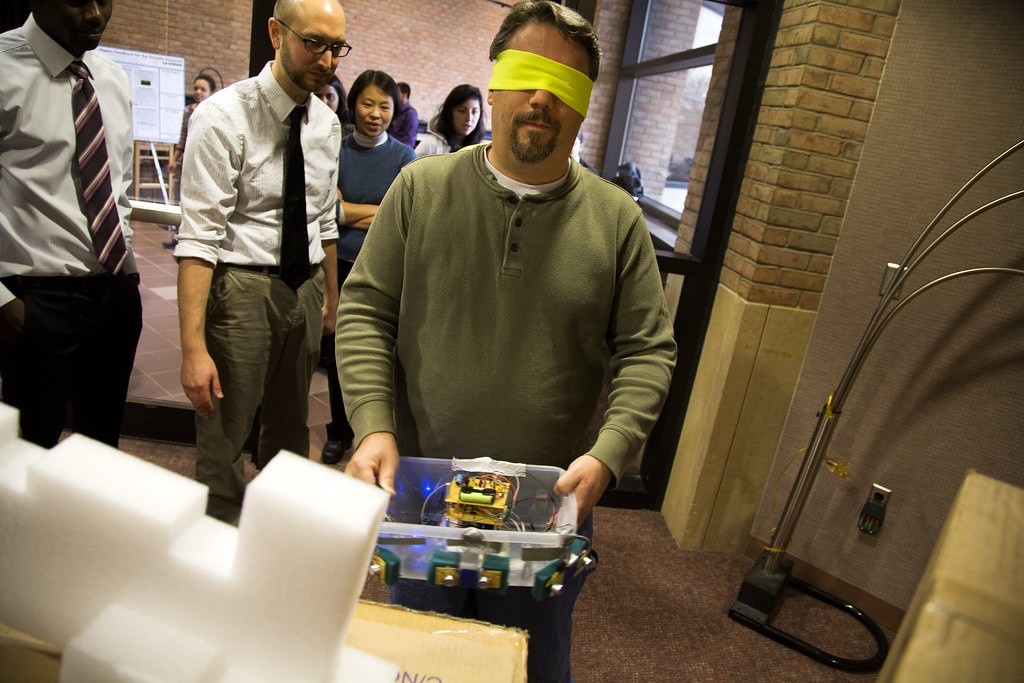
[334,599,530,683]
[873,468,1024,682]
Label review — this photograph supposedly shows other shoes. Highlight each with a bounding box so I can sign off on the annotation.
[163,239,178,247]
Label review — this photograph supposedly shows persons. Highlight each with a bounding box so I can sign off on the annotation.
[335,0,679,683]
[387,82,418,147]
[572,130,597,174]
[0,0,142,450]
[171,0,342,528]
[313,74,348,137]
[162,74,216,249]
[320,69,416,464]
[414,84,484,158]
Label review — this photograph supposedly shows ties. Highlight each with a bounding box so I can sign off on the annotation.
[280,106,309,292]
[67,60,129,276]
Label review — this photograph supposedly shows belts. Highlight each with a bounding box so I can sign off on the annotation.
[217,261,319,278]
[0,273,139,292]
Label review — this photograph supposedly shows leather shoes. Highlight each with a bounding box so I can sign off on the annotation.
[322,440,346,463]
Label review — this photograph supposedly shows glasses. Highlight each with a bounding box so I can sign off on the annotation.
[278,19,352,57]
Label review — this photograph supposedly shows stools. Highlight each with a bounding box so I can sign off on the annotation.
[133,141,175,206]
